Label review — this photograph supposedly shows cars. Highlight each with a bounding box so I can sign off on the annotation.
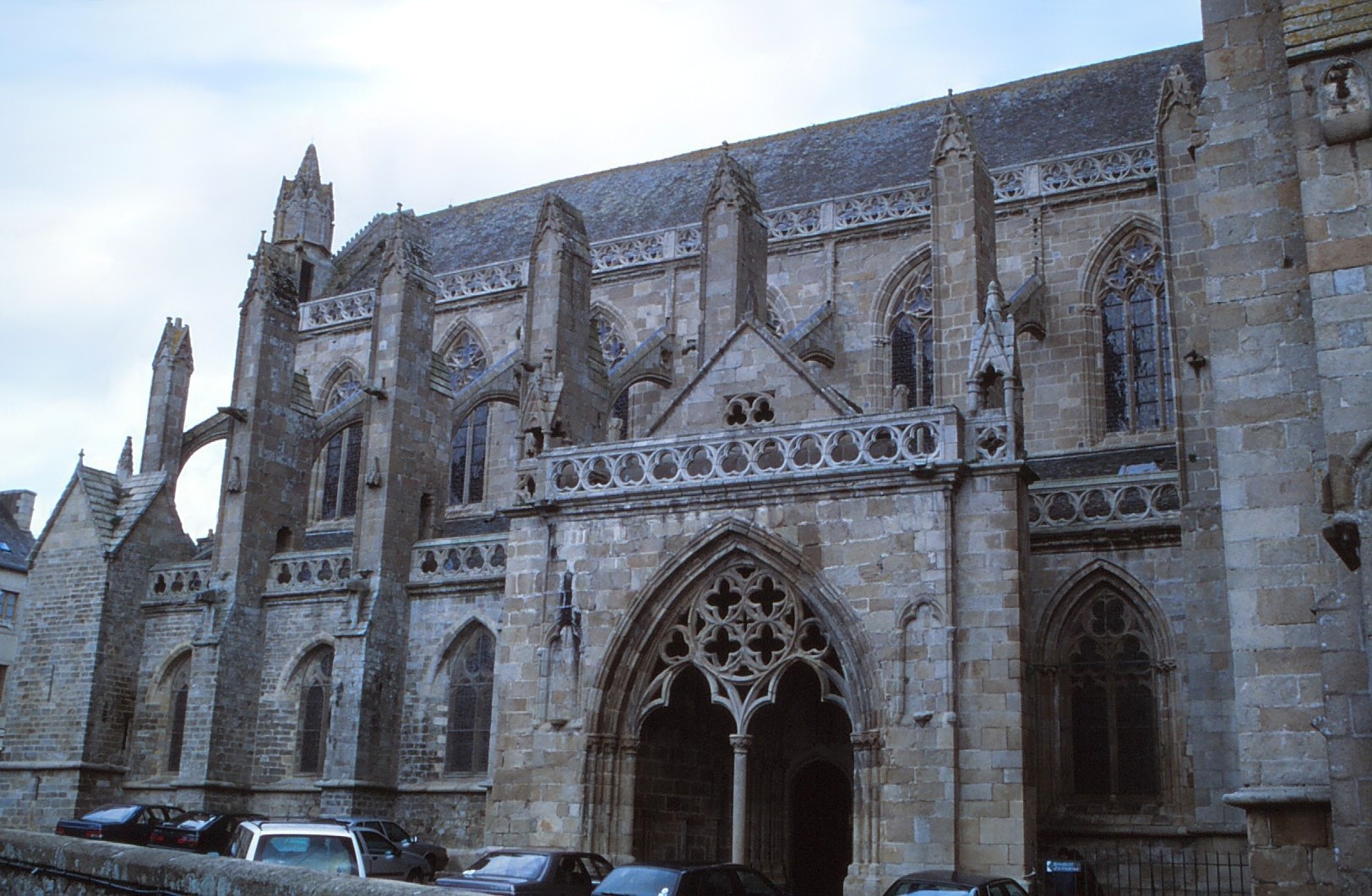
[882,869,1030,896]
[590,861,784,896]
[146,810,271,856]
[434,847,618,896]
[320,814,449,886]
[206,819,366,881]
[54,802,188,848]
